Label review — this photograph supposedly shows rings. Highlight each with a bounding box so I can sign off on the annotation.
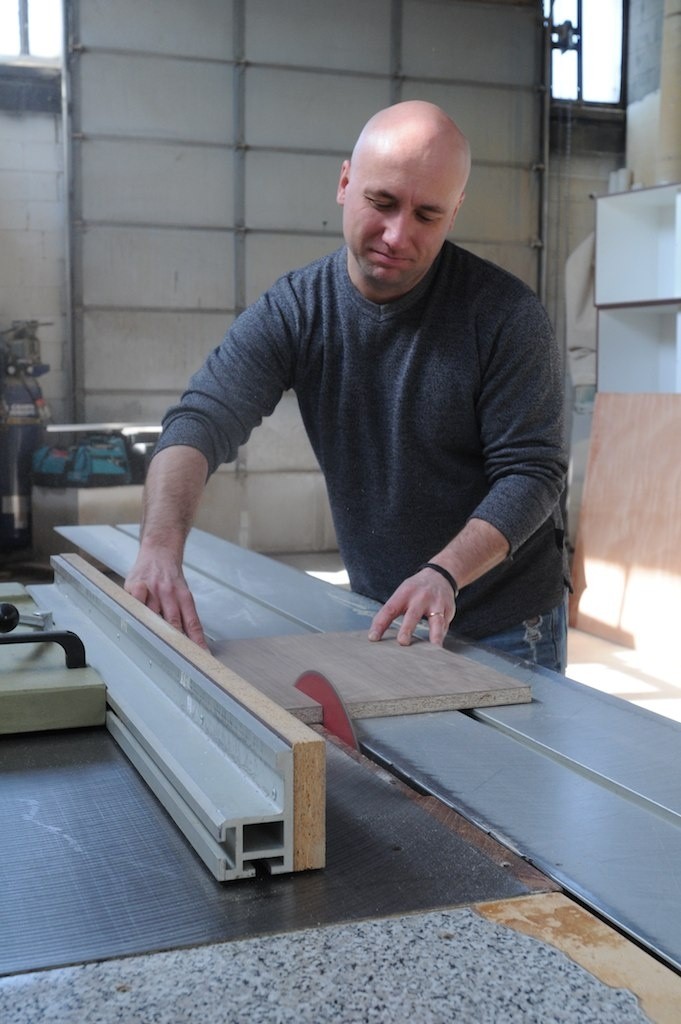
[427,611,444,618]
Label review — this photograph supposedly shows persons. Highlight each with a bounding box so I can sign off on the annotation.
[119,99,576,687]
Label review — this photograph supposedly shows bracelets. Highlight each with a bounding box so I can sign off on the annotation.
[414,561,459,601]
